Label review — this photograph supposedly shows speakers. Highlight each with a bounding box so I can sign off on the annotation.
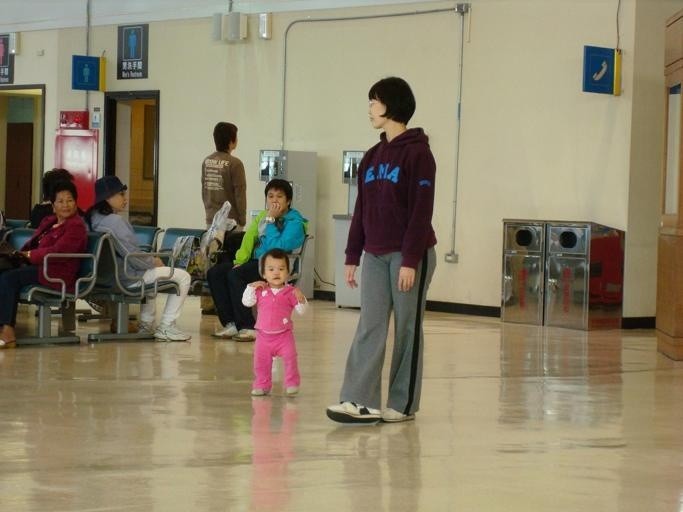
[221,12,248,41]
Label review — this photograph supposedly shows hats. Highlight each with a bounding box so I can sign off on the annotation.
[95,175,127,203]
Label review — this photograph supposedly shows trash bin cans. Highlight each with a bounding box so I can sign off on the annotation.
[500,219,546,326]
[545,220,625,331]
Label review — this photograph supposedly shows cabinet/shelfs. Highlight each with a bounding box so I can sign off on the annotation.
[656,7,683,361]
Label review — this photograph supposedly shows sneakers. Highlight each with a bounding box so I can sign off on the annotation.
[286,387,298,394]
[154,324,191,341]
[0,335,16,348]
[138,322,156,334]
[251,389,264,396]
[211,322,238,336]
[382,408,415,423]
[327,401,382,423]
[232,329,256,341]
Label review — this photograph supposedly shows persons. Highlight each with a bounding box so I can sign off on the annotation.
[206,178,309,342]
[241,248,309,396]
[325,76,438,423]
[78,175,193,341]
[30,168,88,229]
[200,121,247,315]
[0,181,90,349]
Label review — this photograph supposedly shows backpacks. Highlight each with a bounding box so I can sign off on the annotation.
[168,236,204,275]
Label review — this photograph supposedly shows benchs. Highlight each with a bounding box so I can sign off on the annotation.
[129,224,165,253]
[151,227,313,296]
[1,227,107,346]
[90,236,180,341]
[4,219,32,230]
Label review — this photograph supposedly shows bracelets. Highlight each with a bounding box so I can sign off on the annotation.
[27,250,31,259]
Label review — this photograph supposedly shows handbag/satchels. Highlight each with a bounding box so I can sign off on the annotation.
[0,240,22,270]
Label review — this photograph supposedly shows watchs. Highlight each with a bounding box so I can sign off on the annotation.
[265,217,277,223]
